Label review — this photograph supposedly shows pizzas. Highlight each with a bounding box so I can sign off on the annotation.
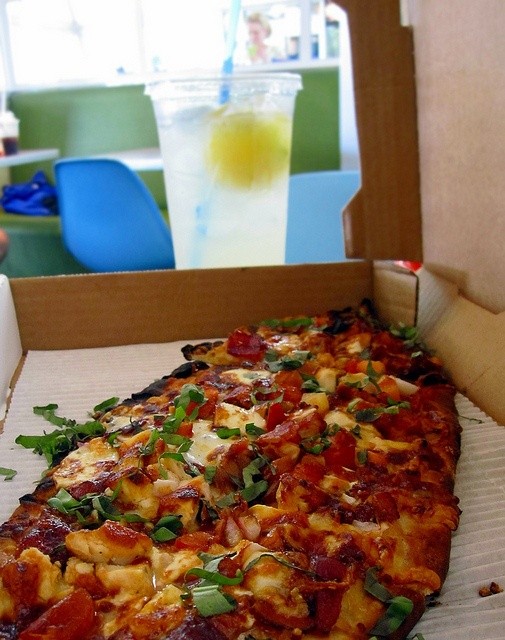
[0,296,463,639]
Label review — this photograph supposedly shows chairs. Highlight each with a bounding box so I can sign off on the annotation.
[286,169,362,264]
[55,157,175,272]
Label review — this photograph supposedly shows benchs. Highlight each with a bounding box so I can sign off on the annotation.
[1,65,339,278]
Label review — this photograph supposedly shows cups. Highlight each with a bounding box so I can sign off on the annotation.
[144,73,304,270]
[0,113,20,154]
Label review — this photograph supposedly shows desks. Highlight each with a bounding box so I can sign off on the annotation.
[102,150,164,171]
[0,149,59,168]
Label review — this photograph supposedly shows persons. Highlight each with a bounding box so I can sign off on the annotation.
[238,16,280,61]
